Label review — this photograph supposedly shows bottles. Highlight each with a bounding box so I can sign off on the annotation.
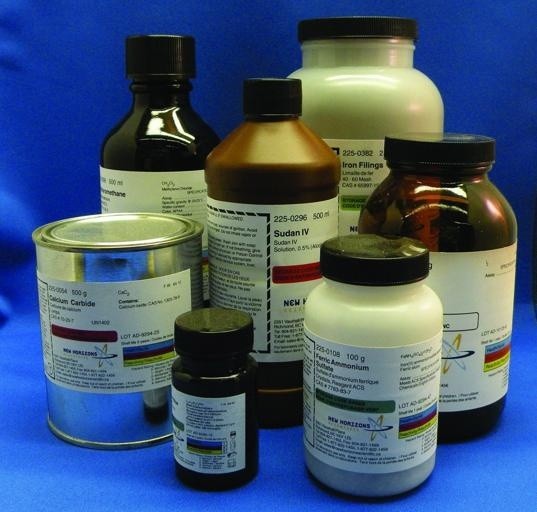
[302,231,444,498]
[99,32,217,307]
[203,78,341,430]
[169,306,260,492]
[357,131,518,446]
[284,18,446,232]
